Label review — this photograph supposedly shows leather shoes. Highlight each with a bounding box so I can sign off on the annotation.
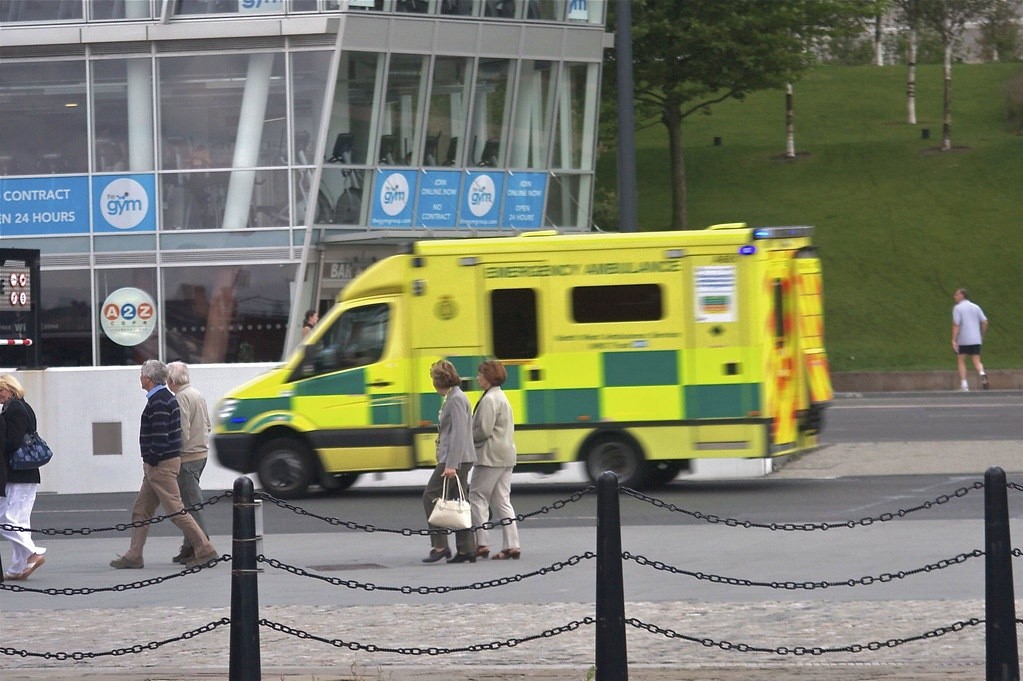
[447,552,476,563]
[422,547,451,562]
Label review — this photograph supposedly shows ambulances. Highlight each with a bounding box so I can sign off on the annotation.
[211,222,833,503]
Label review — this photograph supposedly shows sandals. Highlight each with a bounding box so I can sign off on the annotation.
[474,545,489,558]
[491,548,520,559]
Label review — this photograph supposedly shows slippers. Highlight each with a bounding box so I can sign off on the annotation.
[2,571,22,580]
[20,557,45,580]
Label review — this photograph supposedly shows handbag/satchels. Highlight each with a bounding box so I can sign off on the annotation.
[5,400,53,470]
[428,473,472,530]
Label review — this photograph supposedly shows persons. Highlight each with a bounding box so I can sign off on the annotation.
[108,359,219,569]
[0,373,46,580]
[423,361,478,567]
[192,145,218,217]
[469,360,522,561]
[950,288,989,392]
[302,310,318,341]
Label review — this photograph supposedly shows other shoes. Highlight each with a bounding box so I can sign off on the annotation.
[980,373,990,390]
[110,556,144,569]
[957,387,969,392]
[173,547,219,569]
